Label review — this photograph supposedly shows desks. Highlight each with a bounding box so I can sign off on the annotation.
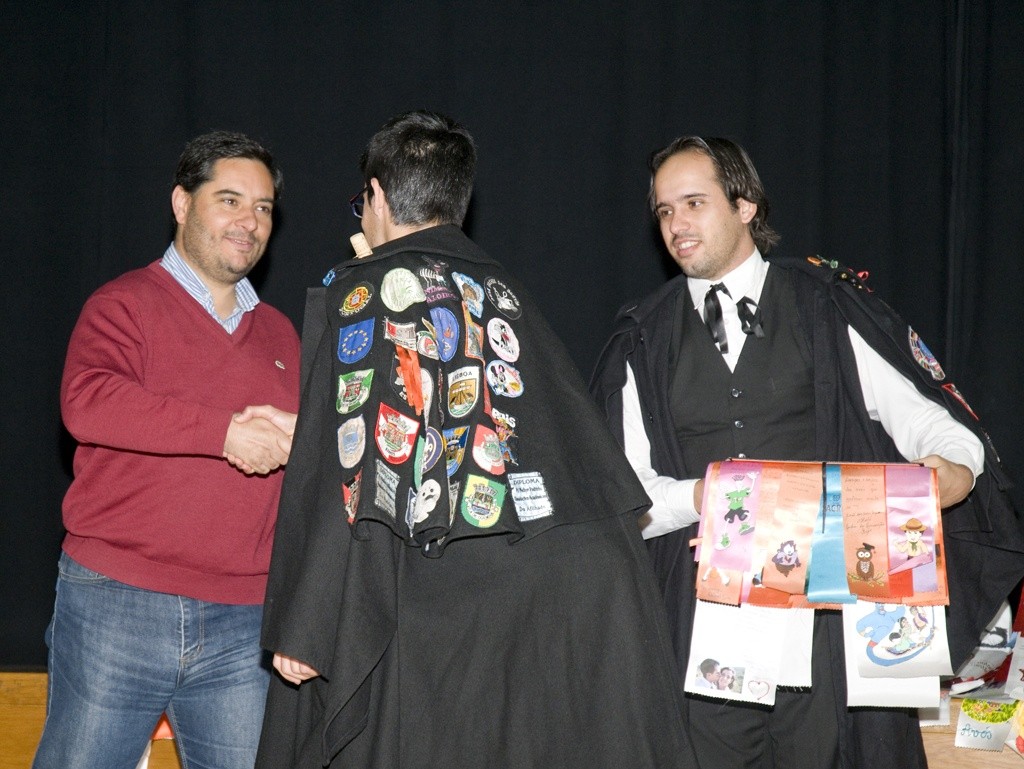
[918,687,1024,769]
[0,670,181,769]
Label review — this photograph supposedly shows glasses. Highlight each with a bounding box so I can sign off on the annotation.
[349,187,370,218]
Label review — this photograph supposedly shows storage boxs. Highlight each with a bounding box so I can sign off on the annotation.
[953,697,1021,753]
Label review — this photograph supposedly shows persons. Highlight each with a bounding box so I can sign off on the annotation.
[591,133,984,769]
[255,113,697,769]
[697,659,735,692]
[30,130,301,769]
